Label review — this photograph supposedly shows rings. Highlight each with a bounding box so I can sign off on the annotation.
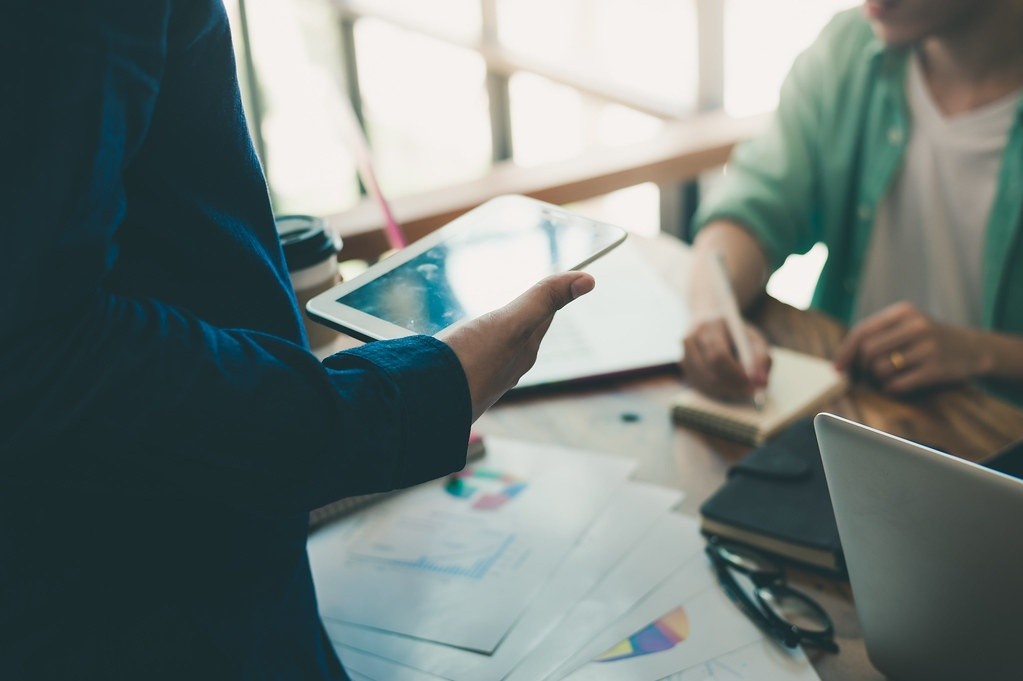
[889,352,905,369]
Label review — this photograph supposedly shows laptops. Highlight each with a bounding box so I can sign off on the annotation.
[813,414,1023,681]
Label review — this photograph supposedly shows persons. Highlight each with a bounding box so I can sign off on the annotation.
[680,0,1023,480]
[0,0,595,681]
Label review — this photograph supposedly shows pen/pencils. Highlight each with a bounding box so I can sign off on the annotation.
[705,253,764,411]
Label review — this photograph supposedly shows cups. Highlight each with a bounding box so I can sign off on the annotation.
[273,214,343,350]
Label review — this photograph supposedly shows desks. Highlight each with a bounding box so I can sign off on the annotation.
[297,283,1023,680]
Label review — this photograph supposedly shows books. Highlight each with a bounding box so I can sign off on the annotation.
[699,417,947,580]
[672,346,852,445]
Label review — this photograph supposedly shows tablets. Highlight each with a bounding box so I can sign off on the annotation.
[305,193,630,344]
